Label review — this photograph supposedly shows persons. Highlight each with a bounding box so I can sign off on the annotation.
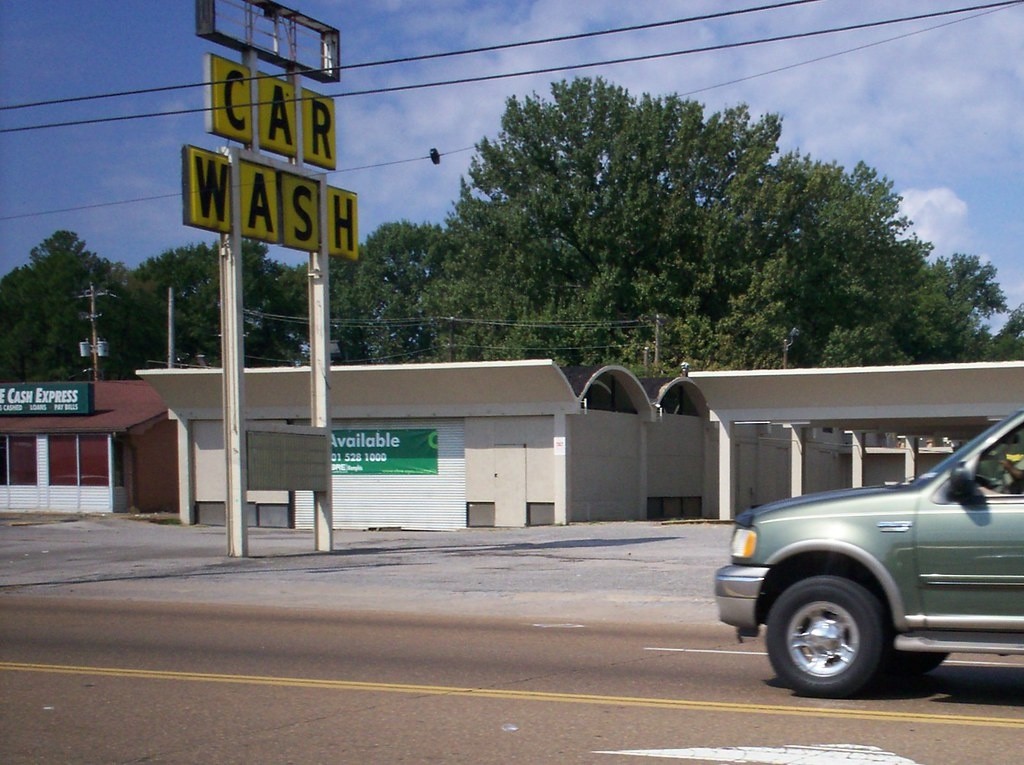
[998,458,1024,482]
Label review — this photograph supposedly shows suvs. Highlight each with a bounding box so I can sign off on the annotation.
[713,409,1024,702]
